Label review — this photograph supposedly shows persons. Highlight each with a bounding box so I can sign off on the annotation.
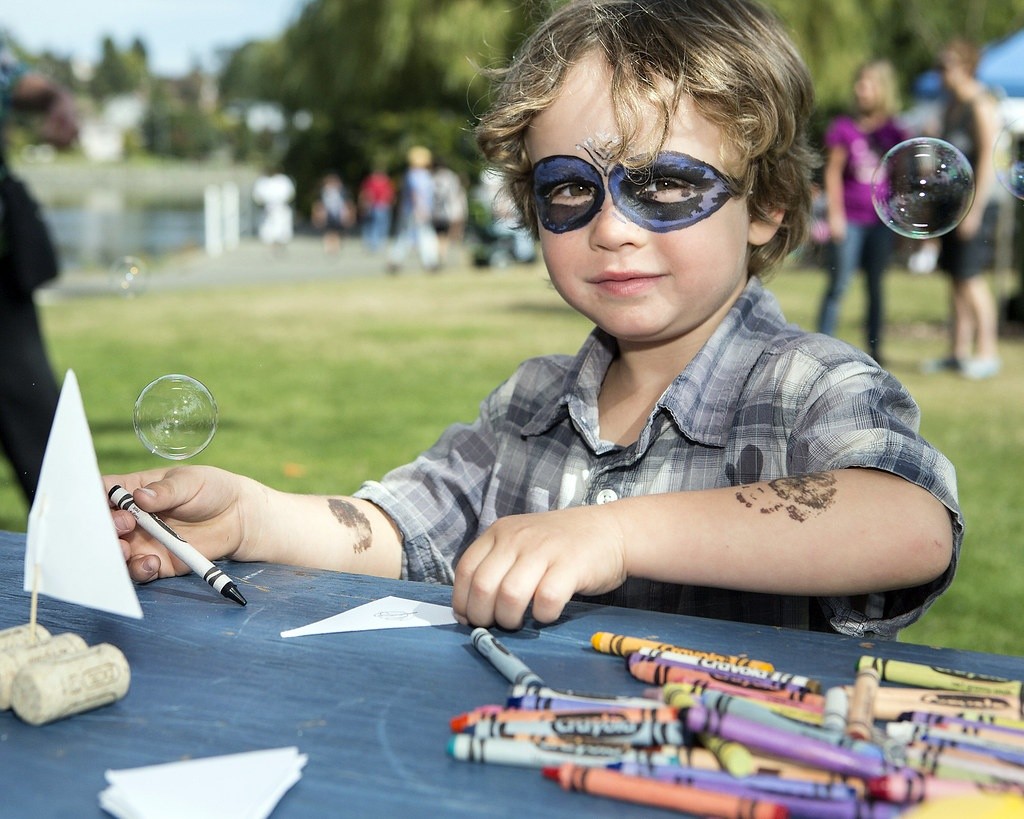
[928,39,1012,379]
[2,152,60,507]
[814,61,922,368]
[100,0,965,637]
[316,138,541,275]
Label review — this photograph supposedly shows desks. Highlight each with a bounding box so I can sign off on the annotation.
[0,527,1024,819]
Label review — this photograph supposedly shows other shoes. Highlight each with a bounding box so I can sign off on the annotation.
[961,354,1001,379]
[920,355,962,376]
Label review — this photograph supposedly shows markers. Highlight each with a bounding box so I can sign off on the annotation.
[106,484,249,609]
[446,625,1024,819]
